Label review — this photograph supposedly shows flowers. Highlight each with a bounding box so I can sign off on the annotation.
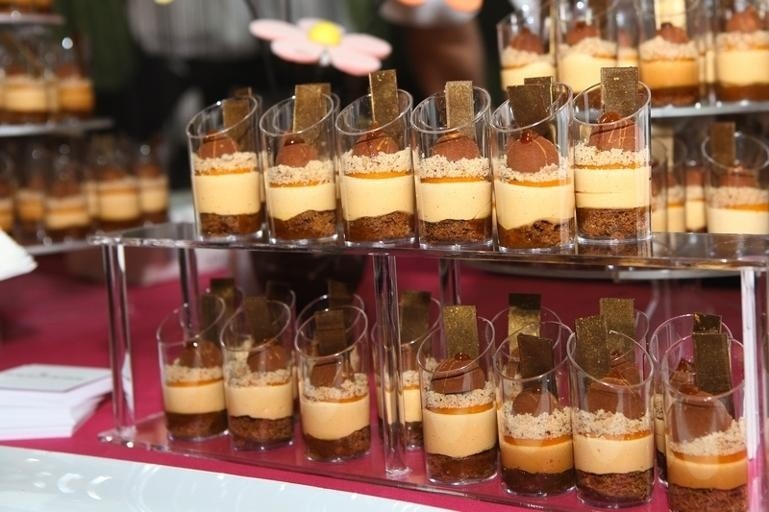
[249,17,392,76]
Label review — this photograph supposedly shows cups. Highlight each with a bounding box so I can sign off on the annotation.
[487,3,768,108]
[0,32,168,249]
[154,284,767,512]
[156,81,767,269]
